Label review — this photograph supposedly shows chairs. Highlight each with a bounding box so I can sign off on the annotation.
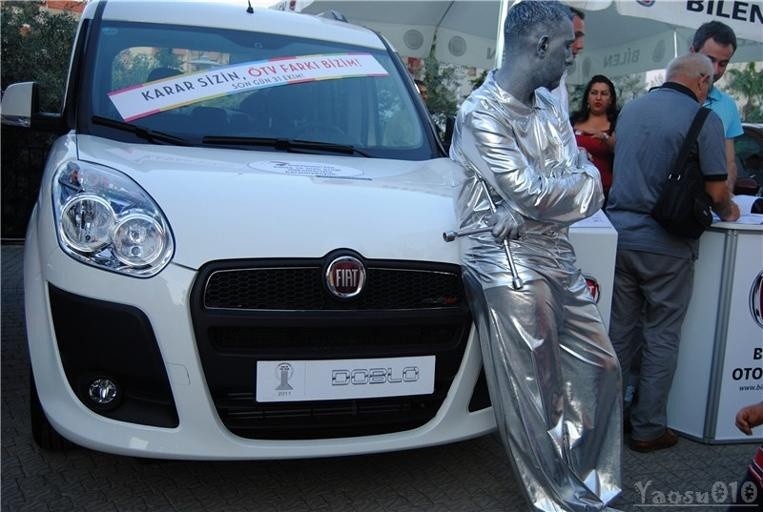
[188,107,228,137]
[146,67,183,85]
[265,87,320,124]
[1,81,41,133]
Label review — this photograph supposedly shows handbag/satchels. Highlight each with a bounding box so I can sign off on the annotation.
[654,156,712,236]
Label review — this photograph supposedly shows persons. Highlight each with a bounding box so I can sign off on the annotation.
[447,1,630,511]
[385,78,429,149]
[687,19,745,202]
[568,75,623,212]
[603,52,742,454]
[727,397,762,512]
[546,3,585,117]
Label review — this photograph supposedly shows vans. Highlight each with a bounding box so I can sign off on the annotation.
[1,0,499,463]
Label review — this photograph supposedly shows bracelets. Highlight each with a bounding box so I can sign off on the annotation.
[602,130,608,142]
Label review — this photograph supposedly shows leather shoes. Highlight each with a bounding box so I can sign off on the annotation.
[630,428,677,451]
